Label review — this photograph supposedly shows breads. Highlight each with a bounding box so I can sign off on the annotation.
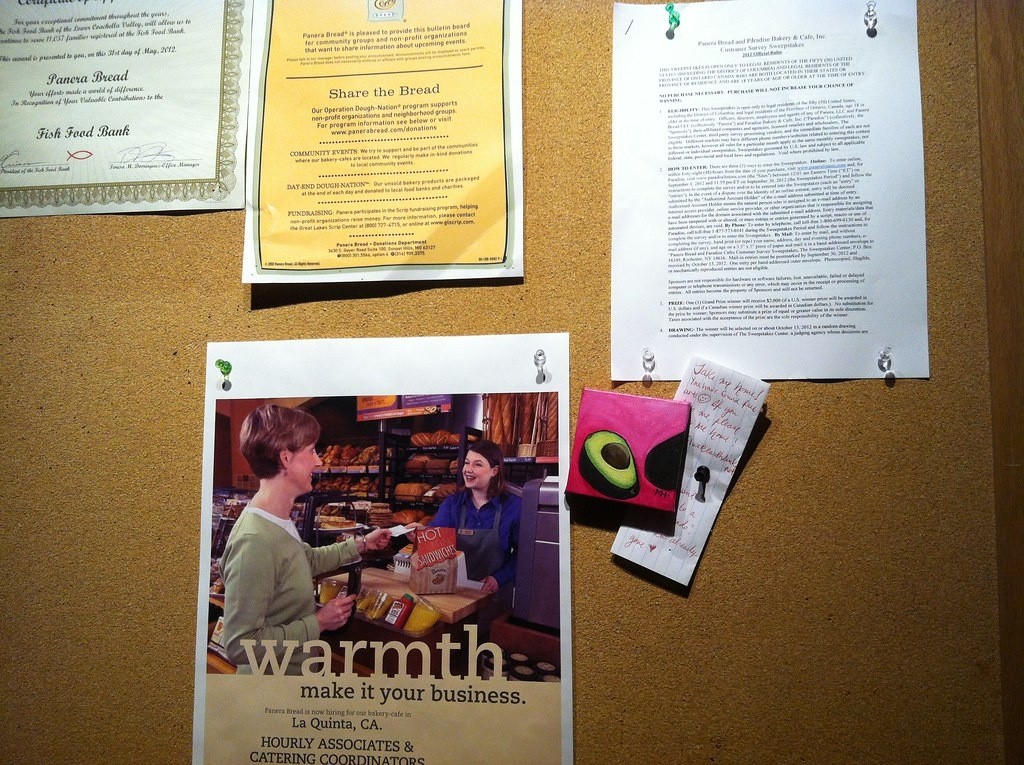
[484,392,559,443]
[209,430,476,594]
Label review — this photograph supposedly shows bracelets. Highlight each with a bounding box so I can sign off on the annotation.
[363,536,368,551]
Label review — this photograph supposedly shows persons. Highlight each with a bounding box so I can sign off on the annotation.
[405,439,523,664]
[220,404,392,675]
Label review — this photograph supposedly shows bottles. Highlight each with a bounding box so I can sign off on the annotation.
[384,592,414,629]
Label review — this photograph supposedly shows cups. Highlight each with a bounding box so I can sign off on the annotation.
[403,595,444,634]
[320,578,392,619]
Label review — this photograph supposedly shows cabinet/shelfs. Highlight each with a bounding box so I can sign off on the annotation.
[314,418,485,542]
